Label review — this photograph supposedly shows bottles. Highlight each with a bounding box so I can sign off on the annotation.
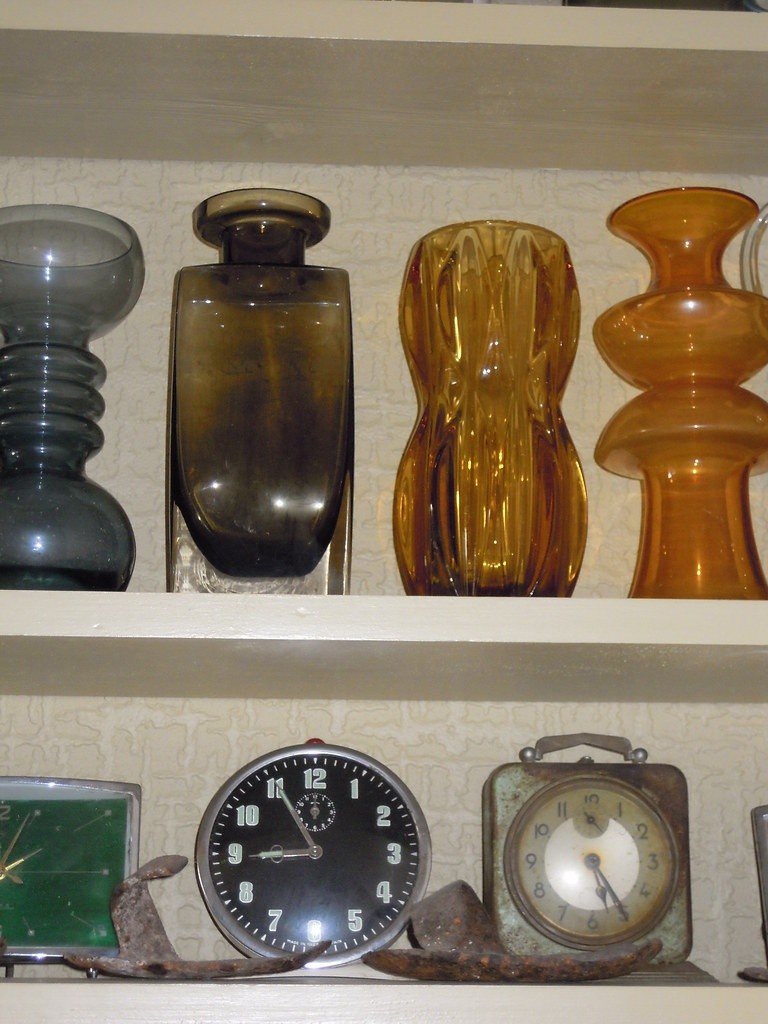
[0,204,146,591]
[594,187,768,601]
[153,187,355,594]
[392,220,589,599]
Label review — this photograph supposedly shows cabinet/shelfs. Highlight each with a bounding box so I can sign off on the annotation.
[0,0,768,1024]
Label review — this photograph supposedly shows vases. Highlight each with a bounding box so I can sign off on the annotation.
[165,187,356,595]
[592,186,768,601]
[392,219,590,599]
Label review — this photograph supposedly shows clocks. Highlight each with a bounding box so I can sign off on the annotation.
[194,737,434,970]
[0,775,142,979]
[481,732,693,966]
